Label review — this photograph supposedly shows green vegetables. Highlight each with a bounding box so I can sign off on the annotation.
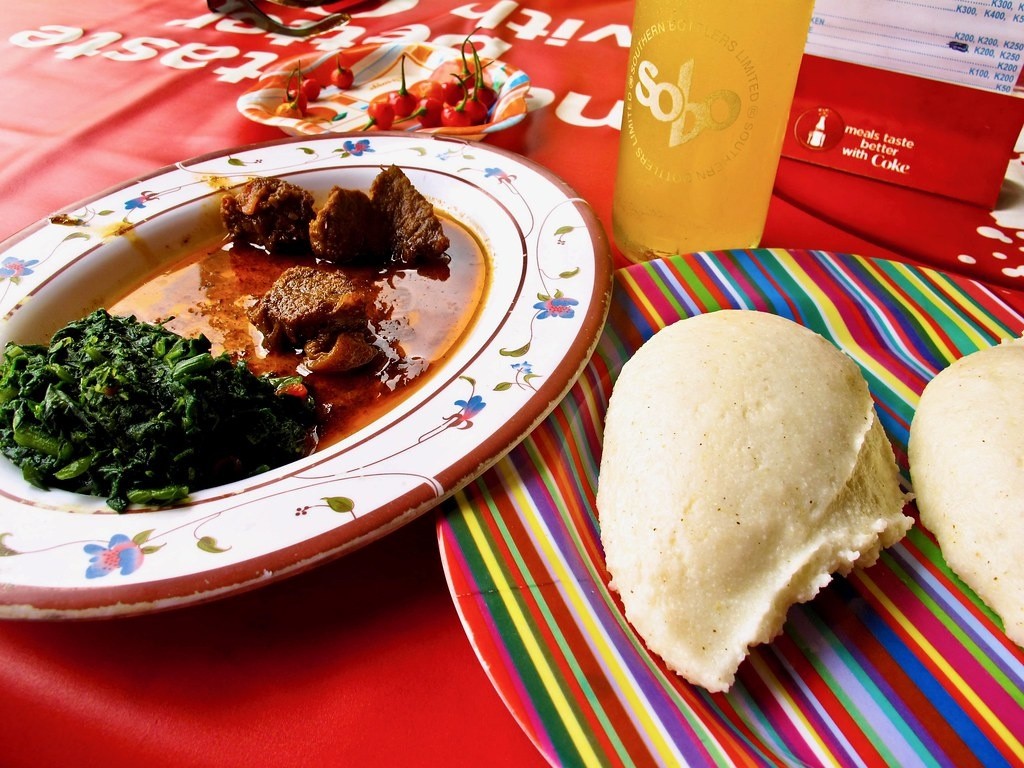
[0,306,330,514]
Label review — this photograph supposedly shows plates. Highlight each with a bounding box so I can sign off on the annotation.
[235,40,531,143]
[0,131,615,619]
[437,247,1024,768]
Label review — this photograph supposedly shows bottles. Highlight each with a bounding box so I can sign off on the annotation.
[611,0,815,266]
